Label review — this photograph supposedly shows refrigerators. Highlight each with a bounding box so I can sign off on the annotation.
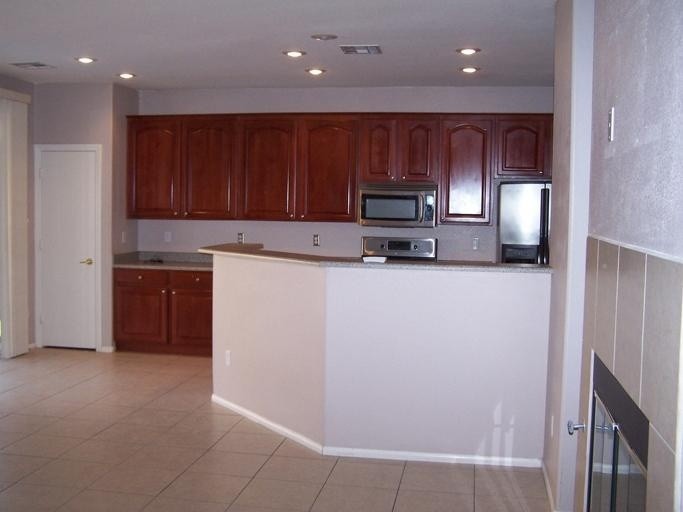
[496,182,552,266]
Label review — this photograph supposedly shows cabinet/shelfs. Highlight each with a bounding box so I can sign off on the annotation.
[494,113,553,179]
[111,267,212,357]
[437,113,494,226]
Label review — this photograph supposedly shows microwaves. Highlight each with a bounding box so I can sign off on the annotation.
[355,182,437,229]
